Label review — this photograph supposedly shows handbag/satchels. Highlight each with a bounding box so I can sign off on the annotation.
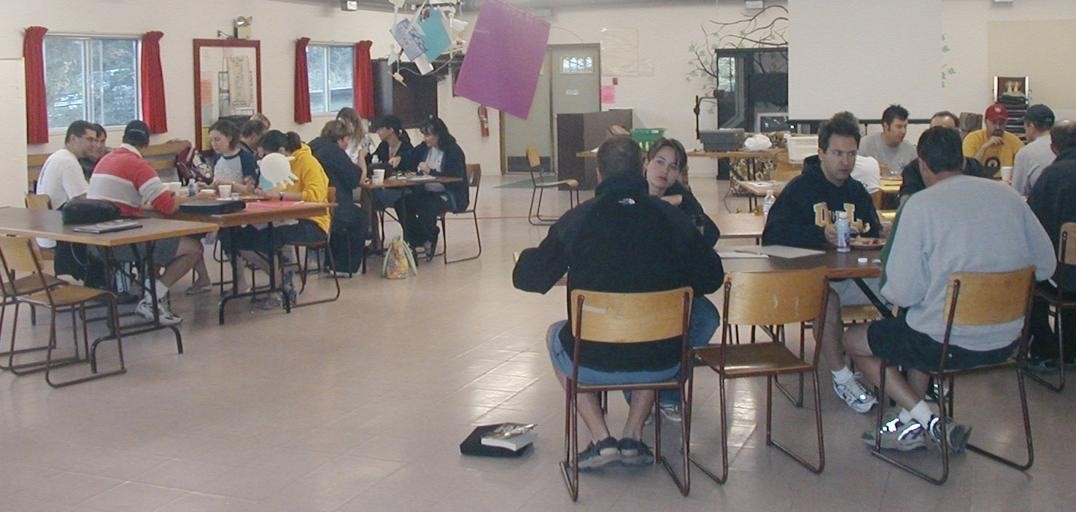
[61,197,118,226]
[382,236,419,281]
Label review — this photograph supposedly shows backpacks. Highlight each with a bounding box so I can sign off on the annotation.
[175,145,214,187]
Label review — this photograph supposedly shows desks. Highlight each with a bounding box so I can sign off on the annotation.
[358,176,464,258]
[513,244,897,289]
[573,150,780,214]
[0,205,219,375]
[163,197,338,326]
[736,176,903,209]
[705,209,895,245]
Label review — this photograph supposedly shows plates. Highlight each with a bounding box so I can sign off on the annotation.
[200,190,216,193]
[216,198,237,201]
[408,177,436,181]
[849,238,887,250]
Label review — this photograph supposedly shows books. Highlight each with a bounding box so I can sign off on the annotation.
[480,423,538,452]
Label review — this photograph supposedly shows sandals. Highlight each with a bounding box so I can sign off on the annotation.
[571,436,620,468]
[620,436,656,468]
[424,233,438,263]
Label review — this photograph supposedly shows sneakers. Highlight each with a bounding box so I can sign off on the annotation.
[253,295,281,309]
[133,296,183,327]
[282,269,299,305]
[831,370,879,415]
[1024,352,1058,376]
[860,415,927,453]
[221,287,251,299]
[929,415,972,463]
[185,284,213,295]
[1063,351,1076,369]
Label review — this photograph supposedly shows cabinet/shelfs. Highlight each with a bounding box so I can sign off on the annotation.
[557,108,632,190]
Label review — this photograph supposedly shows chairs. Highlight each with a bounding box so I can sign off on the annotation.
[679,266,830,484]
[251,188,341,311]
[201,126,213,150]
[1,272,79,371]
[24,194,54,212]
[526,147,579,226]
[869,265,1036,486]
[1,236,126,390]
[414,163,481,265]
[559,286,695,503]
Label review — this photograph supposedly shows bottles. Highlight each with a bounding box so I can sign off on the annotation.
[372,155,379,164]
[188,178,197,197]
[764,190,776,225]
[837,213,850,252]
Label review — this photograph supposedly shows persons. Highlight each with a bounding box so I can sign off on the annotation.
[831,110,882,210]
[900,111,993,207]
[962,102,1026,179]
[1026,119,1076,373]
[855,103,918,178]
[512,134,725,472]
[1010,104,1059,195]
[841,126,1058,456]
[39,112,330,324]
[622,137,720,425]
[306,108,469,275]
[761,119,884,414]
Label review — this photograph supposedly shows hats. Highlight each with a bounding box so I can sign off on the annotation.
[985,103,1009,122]
[1017,103,1055,125]
[124,120,149,141]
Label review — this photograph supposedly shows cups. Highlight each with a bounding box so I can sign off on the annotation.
[1001,167,1012,182]
[373,169,385,184]
[219,185,231,198]
[166,182,181,196]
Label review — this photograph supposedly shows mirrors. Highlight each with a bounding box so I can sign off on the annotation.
[192,39,262,159]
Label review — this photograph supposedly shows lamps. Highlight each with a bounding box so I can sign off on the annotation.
[217,30,234,41]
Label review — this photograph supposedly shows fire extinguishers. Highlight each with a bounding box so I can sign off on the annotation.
[478,103,489,138]
